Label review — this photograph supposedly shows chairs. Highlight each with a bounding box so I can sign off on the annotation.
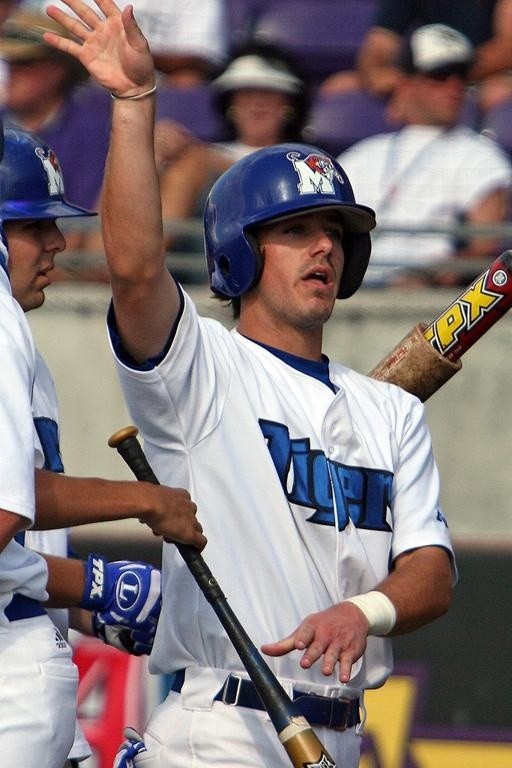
[0,0,512,251]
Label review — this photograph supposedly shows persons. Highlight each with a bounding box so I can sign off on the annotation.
[2,0,510,299]
[30,465,207,551]
[1,122,163,768]
[0,275,82,766]
[39,1,461,768]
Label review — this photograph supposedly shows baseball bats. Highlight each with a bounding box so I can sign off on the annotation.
[367,251,511,404]
[109,426,339,768]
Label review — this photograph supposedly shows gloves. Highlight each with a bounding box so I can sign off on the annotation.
[81,551,163,655]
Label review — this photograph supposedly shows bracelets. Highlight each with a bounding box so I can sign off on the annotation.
[342,589,401,639]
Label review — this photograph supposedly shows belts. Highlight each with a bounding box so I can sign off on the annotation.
[169,667,366,731]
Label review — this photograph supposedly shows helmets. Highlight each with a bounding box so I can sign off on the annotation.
[0,120,100,228]
[202,141,376,301]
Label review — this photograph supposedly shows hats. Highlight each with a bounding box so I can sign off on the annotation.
[400,22,485,78]
[208,50,302,96]
